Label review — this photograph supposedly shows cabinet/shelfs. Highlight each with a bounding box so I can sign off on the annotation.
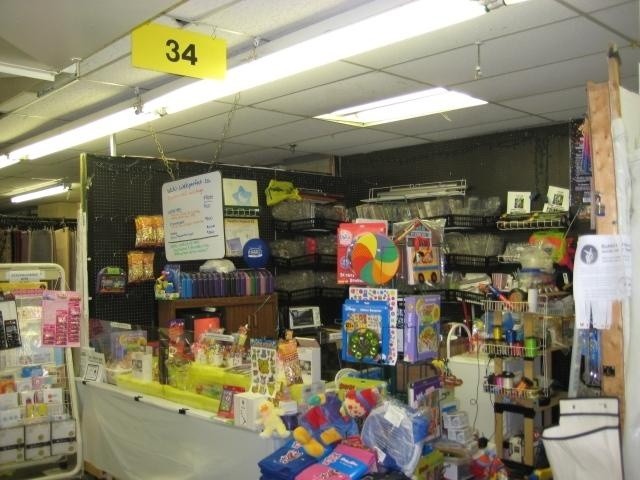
[0,263,85,480]
[85,178,594,479]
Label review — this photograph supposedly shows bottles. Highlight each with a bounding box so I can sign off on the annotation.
[181,268,274,298]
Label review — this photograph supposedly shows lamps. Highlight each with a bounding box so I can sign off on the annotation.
[9,182,72,206]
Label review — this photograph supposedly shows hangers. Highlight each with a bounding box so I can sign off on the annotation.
[1,214,77,231]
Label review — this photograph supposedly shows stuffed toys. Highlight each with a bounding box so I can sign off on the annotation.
[253,400,290,439]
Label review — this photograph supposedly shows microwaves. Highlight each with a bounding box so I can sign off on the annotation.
[284,306,322,330]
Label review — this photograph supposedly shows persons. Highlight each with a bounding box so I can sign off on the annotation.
[290,386,384,459]
